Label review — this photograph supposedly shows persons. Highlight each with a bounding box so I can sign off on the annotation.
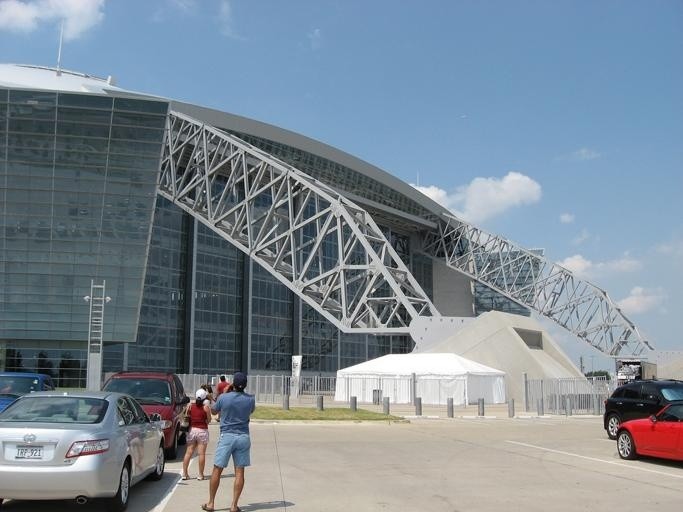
[215,376,230,422]
[181,389,211,480]
[201,372,255,512]
[201,383,213,405]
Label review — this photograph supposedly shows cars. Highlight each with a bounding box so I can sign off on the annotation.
[0,372,79,420]
[616,400,682,464]
[0,388,166,507]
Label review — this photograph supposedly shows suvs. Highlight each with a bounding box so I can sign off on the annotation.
[604,378,683,440]
[87,368,190,459]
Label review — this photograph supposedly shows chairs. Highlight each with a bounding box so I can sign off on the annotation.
[52,414,68,421]
[77,414,99,422]
[16,414,39,419]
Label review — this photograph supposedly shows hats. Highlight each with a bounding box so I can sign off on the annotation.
[234,372,247,388]
[196,389,206,400]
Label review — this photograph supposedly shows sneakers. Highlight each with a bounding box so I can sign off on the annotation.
[182,475,190,479]
[197,477,207,480]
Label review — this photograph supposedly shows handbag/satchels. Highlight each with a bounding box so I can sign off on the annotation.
[180,414,190,432]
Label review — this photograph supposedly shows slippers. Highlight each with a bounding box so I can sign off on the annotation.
[202,504,214,511]
[230,506,241,512]
